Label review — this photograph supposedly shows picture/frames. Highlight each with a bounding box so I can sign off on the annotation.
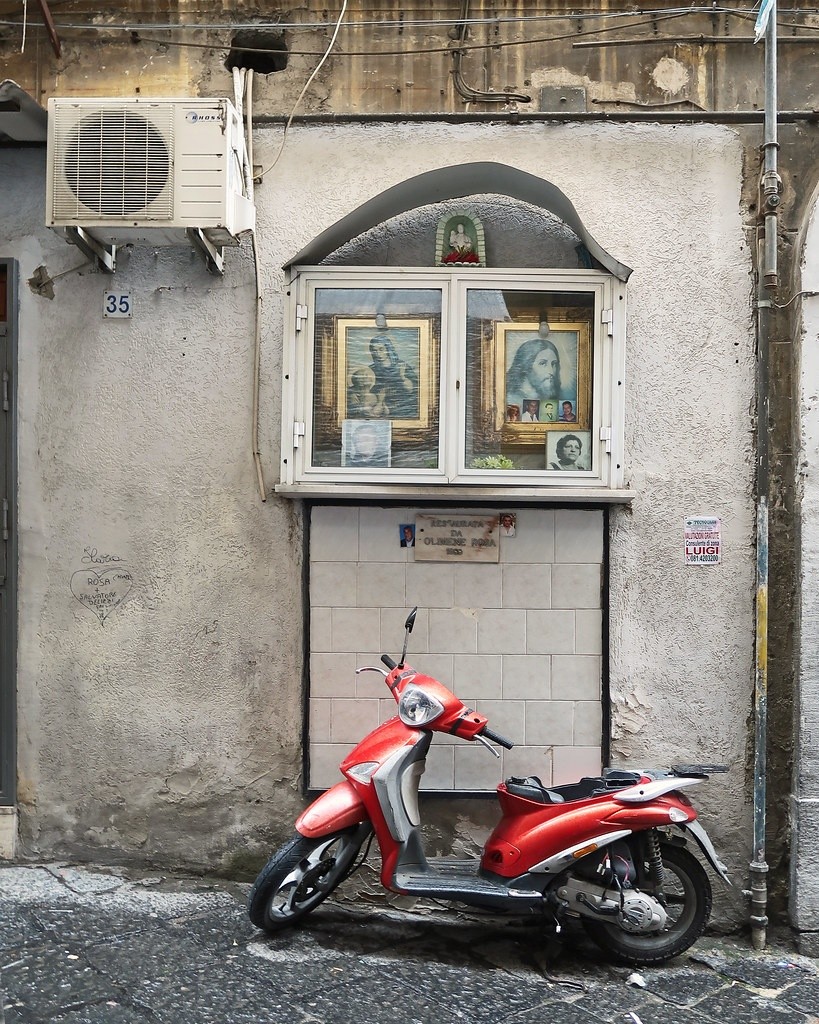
[320,303,438,453]
[470,306,593,454]
[543,429,592,471]
[339,420,391,470]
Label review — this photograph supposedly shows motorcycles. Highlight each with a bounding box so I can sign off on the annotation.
[247,608,734,985]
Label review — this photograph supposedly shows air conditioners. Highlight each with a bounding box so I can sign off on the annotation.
[44,96,256,248]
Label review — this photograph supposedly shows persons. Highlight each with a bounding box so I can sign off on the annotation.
[540,400,559,422]
[345,425,388,467]
[450,224,473,253]
[558,400,577,422]
[499,515,515,538]
[521,399,541,422]
[506,406,520,422]
[401,526,415,548]
[547,434,591,472]
